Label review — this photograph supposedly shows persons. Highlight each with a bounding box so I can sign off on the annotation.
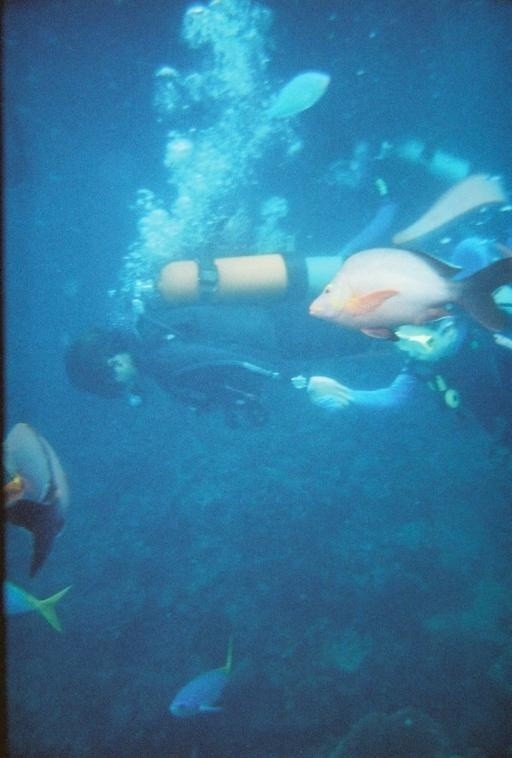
[63,302,411,428]
[309,285,512,454]
